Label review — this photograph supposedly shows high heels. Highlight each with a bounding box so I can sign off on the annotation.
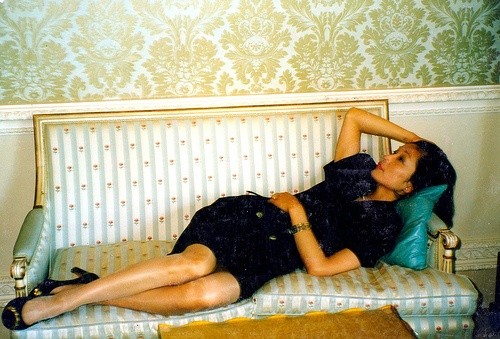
[28,267,100,296]
[1,293,64,330]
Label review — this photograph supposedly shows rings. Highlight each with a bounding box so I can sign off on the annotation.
[271,195,277,199]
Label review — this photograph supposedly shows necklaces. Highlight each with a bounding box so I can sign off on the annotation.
[361,195,368,201]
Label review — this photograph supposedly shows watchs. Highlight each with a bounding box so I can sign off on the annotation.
[288,221,309,235]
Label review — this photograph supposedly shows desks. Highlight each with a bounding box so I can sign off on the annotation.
[152,305,428,339]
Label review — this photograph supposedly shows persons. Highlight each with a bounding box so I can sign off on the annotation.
[1,107,458,331]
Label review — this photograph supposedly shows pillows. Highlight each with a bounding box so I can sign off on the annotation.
[51,241,174,284]
[380,183,448,270]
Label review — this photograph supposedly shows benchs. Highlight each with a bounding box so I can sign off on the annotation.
[9,98,477,339]
